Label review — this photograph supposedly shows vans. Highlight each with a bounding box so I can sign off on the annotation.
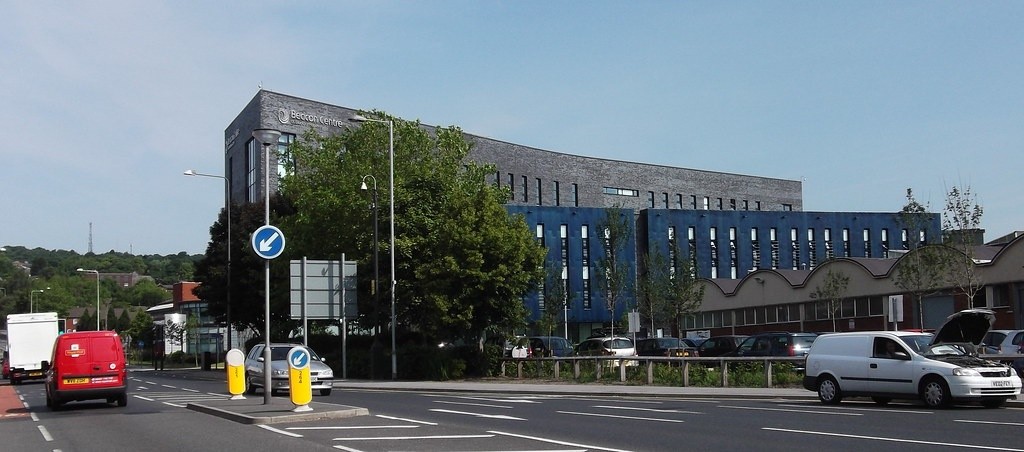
[573,338,639,367]
[803,310,1022,408]
[7,312,59,385]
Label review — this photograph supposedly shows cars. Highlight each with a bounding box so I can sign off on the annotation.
[245,342,333,396]
[698,336,752,357]
[682,339,707,347]
[986,330,1024,355]
[636,338,699,363]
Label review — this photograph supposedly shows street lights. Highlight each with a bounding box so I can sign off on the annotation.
[350,113,399,383]
[77,268,99,331]
[30,290,44,312]
[181,168,232,383]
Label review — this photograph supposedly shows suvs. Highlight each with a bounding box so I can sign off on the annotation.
[512,337,574,359]
[719,332,817,371]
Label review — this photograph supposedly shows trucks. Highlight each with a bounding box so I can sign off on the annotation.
[42,333,127,407]
